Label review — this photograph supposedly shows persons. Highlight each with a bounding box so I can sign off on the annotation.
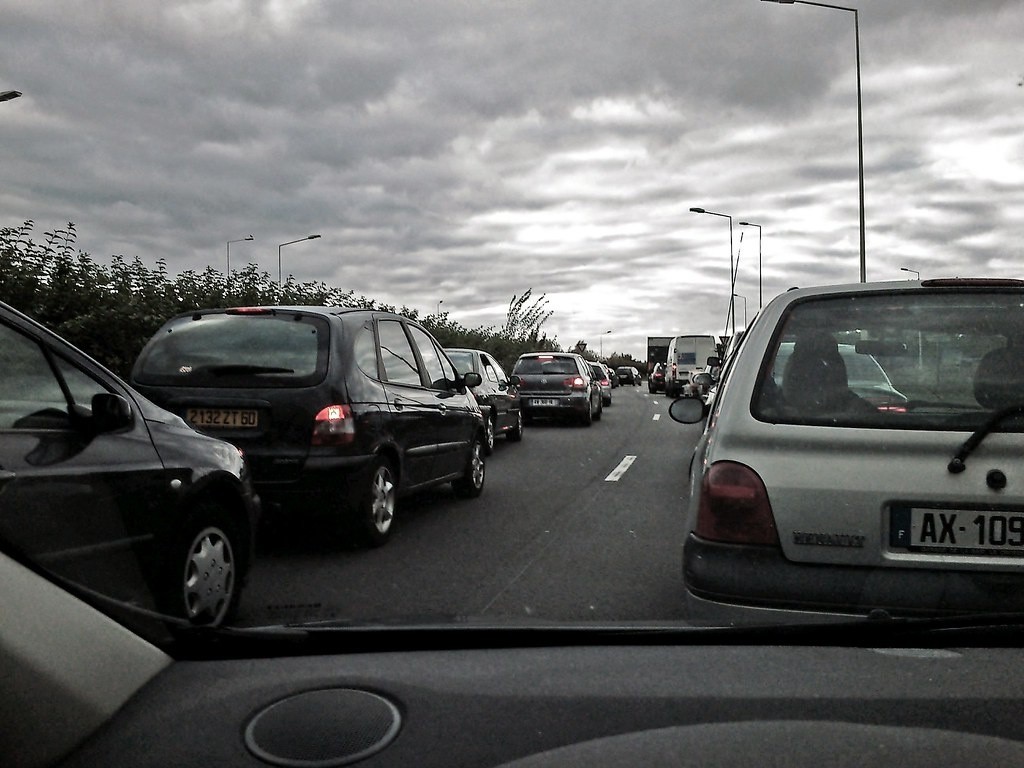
[794,331,880,413]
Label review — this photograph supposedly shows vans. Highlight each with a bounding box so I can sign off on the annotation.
[663,335,717,397]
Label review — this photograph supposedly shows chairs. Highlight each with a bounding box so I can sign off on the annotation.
[761,348,882,415]
[975,347,1024,411]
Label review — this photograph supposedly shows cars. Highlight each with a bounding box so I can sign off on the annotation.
[668,277,1024,632]
[510,352,601,426]
[0,300,260,629]
[130,304,493,547]
[586,359,643,407]
[678,330,904,412]
[647,362,668,394]
[443,348,522,454]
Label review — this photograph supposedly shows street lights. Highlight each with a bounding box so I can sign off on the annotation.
[761,0,868,284]
[689,208,735,335]
[738,222,762,309]
[279,234,321,289]
[600,330,611,363]
[901,268,919,280]
[733,293,747,330]
[227,235,254,277]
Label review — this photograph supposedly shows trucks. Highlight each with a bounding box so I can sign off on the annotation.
[647,337,672,382]
